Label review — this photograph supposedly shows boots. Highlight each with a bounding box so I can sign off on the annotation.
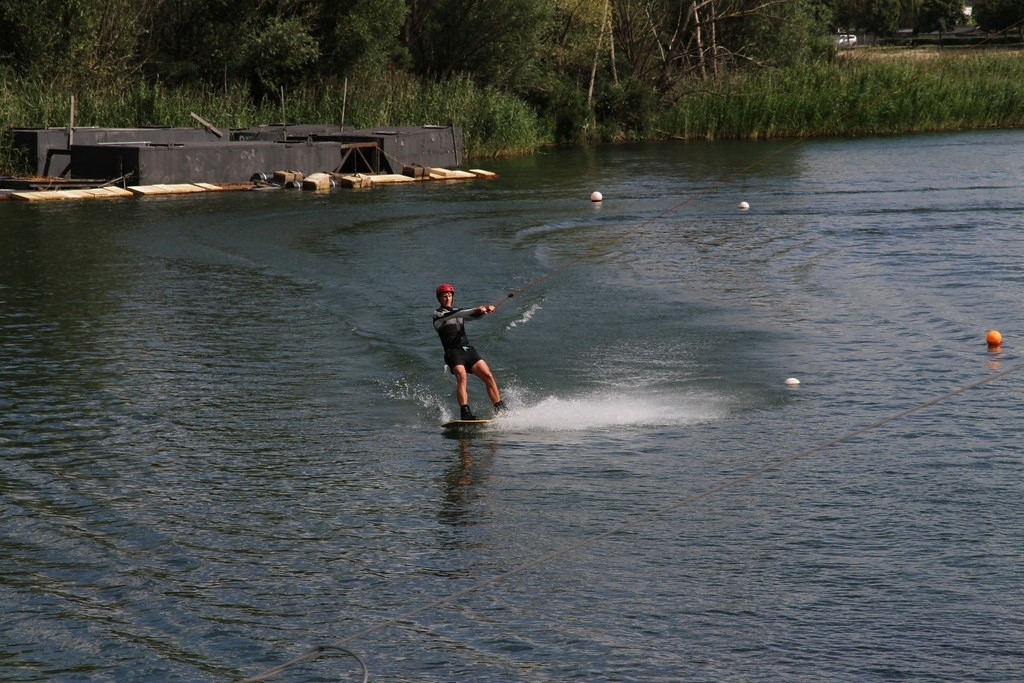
[495,401,510,413]
[460,405,479,420]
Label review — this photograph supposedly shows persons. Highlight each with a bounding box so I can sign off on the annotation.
[433,284,511,421]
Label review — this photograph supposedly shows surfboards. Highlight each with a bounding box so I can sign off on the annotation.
[441,419,493,428]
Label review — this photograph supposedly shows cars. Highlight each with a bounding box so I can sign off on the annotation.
[839,35,857,46]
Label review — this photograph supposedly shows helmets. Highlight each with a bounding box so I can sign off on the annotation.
[436,284,455,298]
